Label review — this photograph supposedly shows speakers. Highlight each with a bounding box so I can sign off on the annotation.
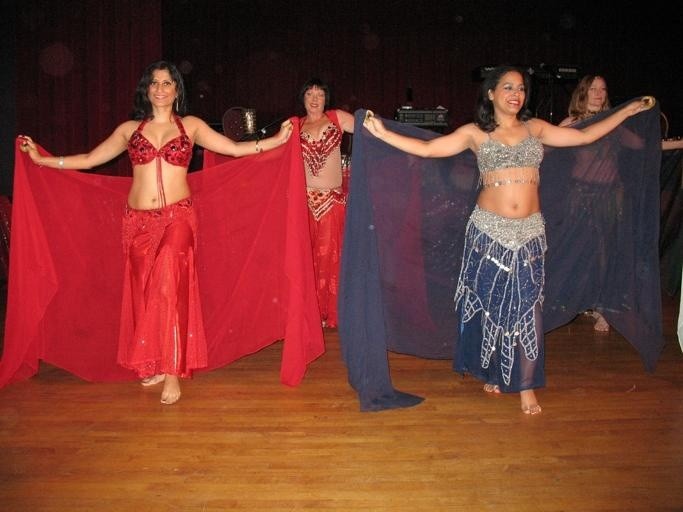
[480,63,579,124]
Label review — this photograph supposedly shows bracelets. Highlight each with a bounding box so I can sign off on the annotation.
[255,137,264,154]
[58,155,64,170]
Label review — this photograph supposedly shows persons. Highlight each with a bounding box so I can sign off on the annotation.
[18,61,293,405]
[282,79,381,331]
[364,59,652,415]
[540,74,683,333]
